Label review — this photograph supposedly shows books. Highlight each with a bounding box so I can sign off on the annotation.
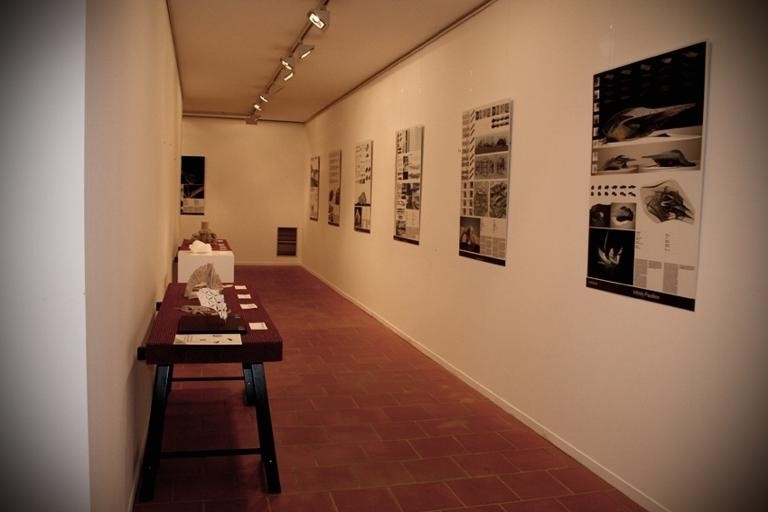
[177,313,247,334]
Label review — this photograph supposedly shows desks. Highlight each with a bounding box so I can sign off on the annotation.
[137,282,287,501]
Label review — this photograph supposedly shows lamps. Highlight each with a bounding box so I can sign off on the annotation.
[278,39,315,70]
[307,2,331,31]
